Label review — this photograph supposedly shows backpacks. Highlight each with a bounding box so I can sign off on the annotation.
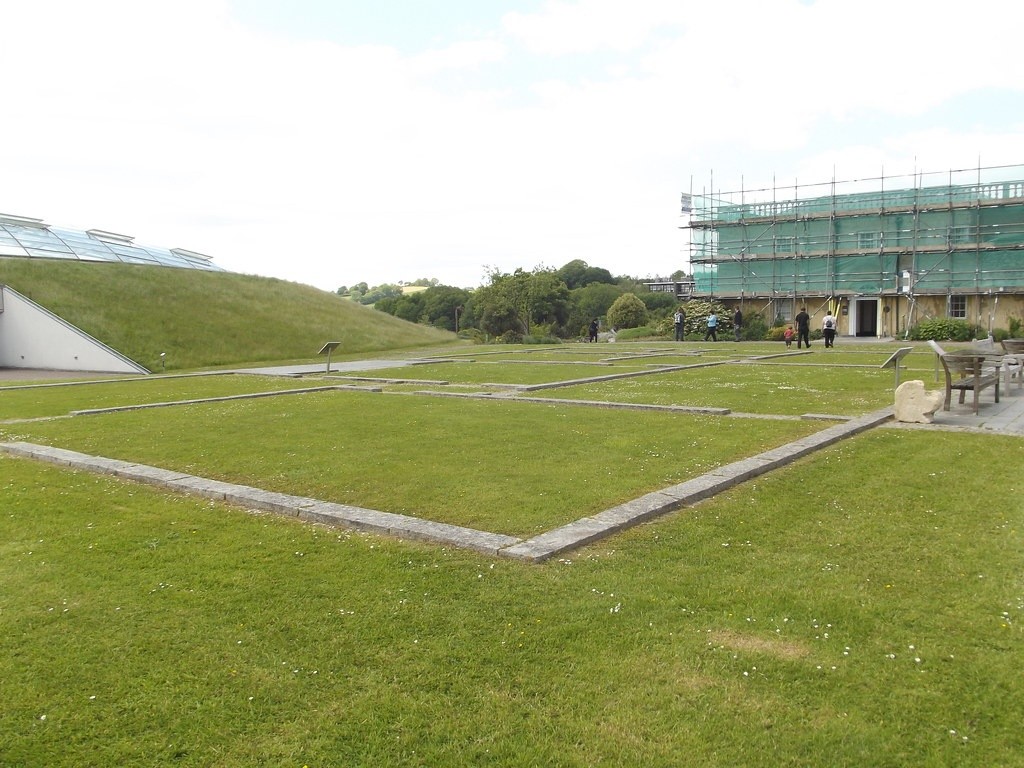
[590,326,595,333]
[675,313,681,324]
[826,317,832,327]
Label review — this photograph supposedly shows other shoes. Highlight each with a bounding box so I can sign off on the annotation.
[798,346,801,348]
[735,341,740,342]
[681,340,684,341]
[807,345,811,348]
[829,343,833,348]
[713,340,717,341]
[826,346,829,348]
[704,338,707,341]
[677,339,678,341]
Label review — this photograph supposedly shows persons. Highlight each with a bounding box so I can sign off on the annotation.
[821,311,835,348]
[733,306,742,342]
[703,311,716,341]
[784,327,795,347]
[674,307,686,341]
[590,318,598,343]
[794,307,811,349]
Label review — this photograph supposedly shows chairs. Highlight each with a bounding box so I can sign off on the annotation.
[1002,340,1024,354]
[972,336,1024,397]
[942,349,1001,414]
